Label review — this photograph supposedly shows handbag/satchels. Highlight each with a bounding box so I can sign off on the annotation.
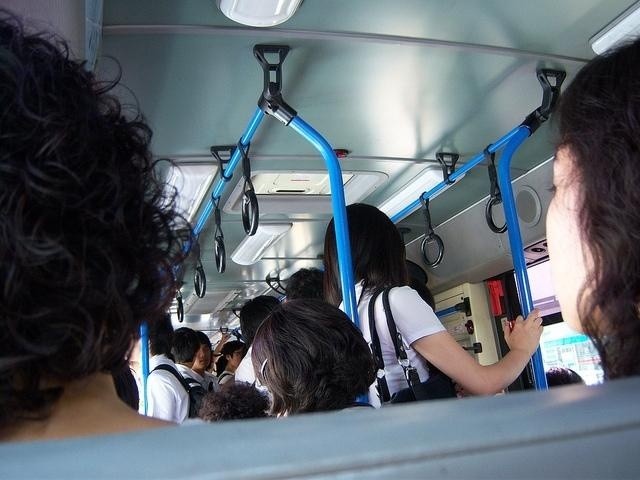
[368,285,458,409]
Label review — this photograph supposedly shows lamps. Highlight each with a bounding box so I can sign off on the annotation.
[160,162,219,230]
[230,223,293,265]
[588,0,640,57]
[377,165,466,224]
[216,0,304,28]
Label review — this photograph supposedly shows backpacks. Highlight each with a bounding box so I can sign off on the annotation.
[149,364,207,419]
